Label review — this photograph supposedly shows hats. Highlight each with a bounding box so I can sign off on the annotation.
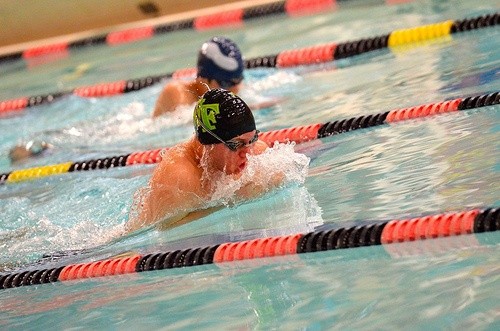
[193,88,256,146]
[198,36,243,78]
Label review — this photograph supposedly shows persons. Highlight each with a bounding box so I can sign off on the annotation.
[7,142,54,161]
[153,35,244,115]
[139,88,270,218]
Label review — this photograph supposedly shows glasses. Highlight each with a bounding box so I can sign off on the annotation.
[199,120,259,151]
[217,77,246,89]
